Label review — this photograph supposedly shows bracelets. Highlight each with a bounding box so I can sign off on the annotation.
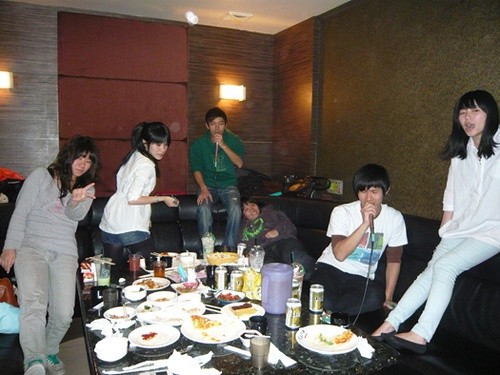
[155,195,158,202]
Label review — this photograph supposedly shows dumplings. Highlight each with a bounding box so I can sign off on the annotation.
[309,284,324,312]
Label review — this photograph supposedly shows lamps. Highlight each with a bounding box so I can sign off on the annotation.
[219,84,246,102]
[0,70,14,90]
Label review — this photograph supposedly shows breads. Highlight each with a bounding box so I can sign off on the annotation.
[232,303,257,317]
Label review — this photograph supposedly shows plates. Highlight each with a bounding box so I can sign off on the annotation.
[137,300,206,327]
[140,252,201,273]
[127,324,181,349]
[295,324,359,355]
[221,302,265,321]
[180,314,246,344]
[215,290,246,302]
[170,279,212,294]
[132,277,171,290]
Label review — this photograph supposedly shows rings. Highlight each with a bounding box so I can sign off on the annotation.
[83,195,85,197]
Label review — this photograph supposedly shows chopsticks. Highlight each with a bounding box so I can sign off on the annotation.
[89,256,115,265]
[123,303,128,319]
[201,303,223,313]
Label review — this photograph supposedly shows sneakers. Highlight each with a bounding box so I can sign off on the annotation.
[46,354,65,375]
[24,359,45,375]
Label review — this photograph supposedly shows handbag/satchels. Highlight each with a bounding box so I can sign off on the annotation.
[282,174,316,200]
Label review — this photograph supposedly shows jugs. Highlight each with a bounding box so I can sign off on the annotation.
[261,262,294,314]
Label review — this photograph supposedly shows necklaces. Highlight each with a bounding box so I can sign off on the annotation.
[55,171,59,189]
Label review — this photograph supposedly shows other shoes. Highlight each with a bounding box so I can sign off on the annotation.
[381,332,426,355]
[369,331,397,342]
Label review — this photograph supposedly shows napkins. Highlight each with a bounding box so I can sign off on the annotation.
[238,329,280,365]
[357,336,375,359]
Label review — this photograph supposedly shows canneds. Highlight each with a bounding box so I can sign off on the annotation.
[230,270,244,292]
[285,298,302,329]
[237,242,246,258]
[292,280,300,299]
[215,266,228,289]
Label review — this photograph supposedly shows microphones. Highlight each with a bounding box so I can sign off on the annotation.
[214,141,220,161]
[368,213,375,241]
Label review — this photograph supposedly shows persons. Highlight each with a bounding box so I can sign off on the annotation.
[98,121,179,257]
[310,163,409,317]
[188,107,242,254]
[370,90,500,353]
[0,134,98,375]
[235,195,316,280]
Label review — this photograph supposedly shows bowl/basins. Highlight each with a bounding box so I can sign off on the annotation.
[103,306,137,324]
[146,290,177,308]
[122,285,146,301]
[179,252,197,266]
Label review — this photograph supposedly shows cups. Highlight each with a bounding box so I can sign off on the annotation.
[154,261,166,278]
[205,264,216,289]
[250,337,270,370]
[290,263,305,300]
[249,316,268,336]
[102,289,119,311]
[129,254,140,273]
[202,232,215,262]
[240,330,262,348]
[250,245,265,273]
[95,257,112,288]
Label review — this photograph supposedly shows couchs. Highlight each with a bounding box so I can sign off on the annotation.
[0,193,500,375]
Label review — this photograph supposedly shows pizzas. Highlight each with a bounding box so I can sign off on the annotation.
[191,315,222,329]
[334,331,352,343]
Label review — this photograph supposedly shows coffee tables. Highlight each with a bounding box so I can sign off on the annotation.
[75,246,426,375]
